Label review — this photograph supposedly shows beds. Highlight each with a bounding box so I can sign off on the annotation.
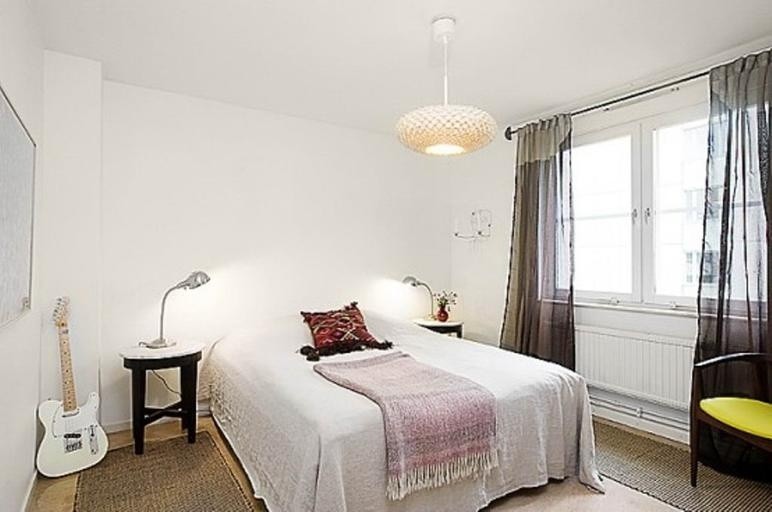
[197,314,604,512]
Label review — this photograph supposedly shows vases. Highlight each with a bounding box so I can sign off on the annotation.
[437,306,449,322]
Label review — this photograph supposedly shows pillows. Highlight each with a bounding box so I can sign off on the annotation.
[300,299,378,347]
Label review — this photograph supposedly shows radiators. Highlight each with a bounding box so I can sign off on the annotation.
[574,323,696,414]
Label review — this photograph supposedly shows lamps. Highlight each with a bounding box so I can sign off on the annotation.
[138,271,211,349]
[402,276,436,320]
[395,17,497,156]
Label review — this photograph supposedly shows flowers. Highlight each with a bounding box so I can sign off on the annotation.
[433,290,459,311]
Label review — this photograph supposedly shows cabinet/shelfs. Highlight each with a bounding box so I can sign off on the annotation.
[411,318,462,339]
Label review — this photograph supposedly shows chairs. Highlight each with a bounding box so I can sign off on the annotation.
[690,353,772,487]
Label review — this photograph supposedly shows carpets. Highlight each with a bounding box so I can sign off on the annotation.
[72,430,252,512]
[591,420,772,512]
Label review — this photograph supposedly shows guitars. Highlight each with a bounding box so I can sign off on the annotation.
[36,296,108,478]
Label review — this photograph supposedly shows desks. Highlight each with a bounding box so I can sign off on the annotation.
[123,342,202,456]
